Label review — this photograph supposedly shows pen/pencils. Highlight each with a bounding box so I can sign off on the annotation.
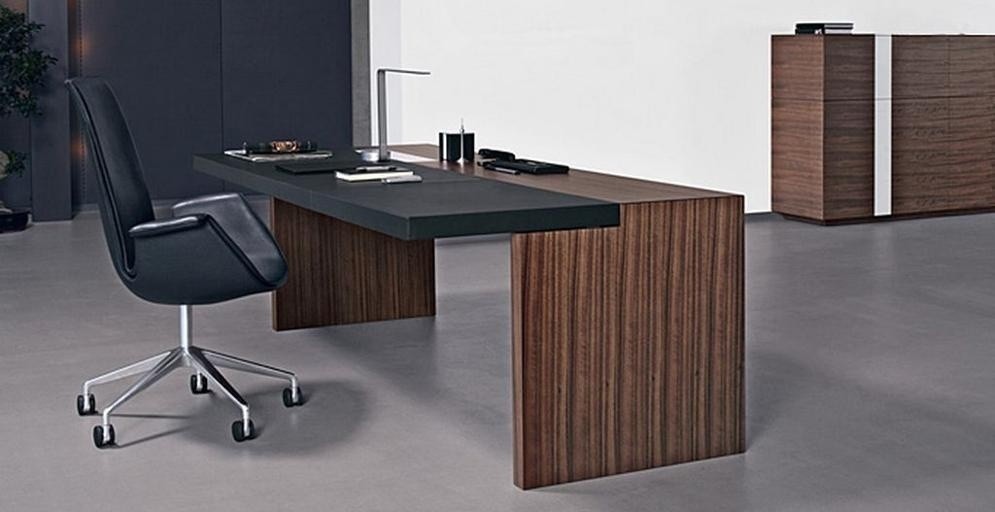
[355,165,396,172]
[488,167,518,175]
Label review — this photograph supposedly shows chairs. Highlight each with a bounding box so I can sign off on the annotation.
[65,75,304,449]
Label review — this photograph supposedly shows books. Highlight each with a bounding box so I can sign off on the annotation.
[795,23,855,35]
[334,165,414,183]
[491,159,569,174]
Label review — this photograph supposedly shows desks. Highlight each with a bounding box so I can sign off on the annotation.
[190,141,747,489]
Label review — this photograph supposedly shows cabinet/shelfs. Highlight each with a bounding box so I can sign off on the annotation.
[772,33,995,226]
